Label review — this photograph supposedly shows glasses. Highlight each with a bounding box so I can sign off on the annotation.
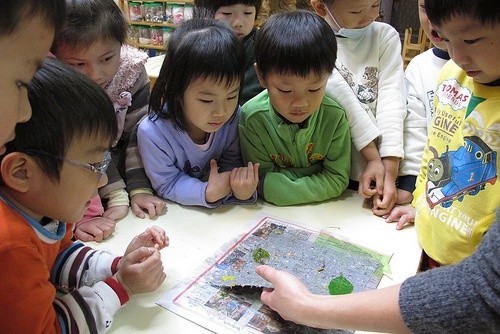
[29,148,113,176]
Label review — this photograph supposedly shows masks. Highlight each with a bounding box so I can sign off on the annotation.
[321,3,379,40]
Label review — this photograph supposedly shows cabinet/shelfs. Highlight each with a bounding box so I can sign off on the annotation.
[117,0,196,57]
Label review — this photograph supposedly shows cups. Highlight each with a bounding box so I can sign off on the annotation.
[128,2,193,46]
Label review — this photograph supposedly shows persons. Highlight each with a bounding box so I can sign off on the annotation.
[193,0,500,269]
[254,204,500,334]
[0,0,169,334]
[137,18,260,208]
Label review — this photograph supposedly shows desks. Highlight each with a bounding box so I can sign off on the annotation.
[72,187,425,334]
